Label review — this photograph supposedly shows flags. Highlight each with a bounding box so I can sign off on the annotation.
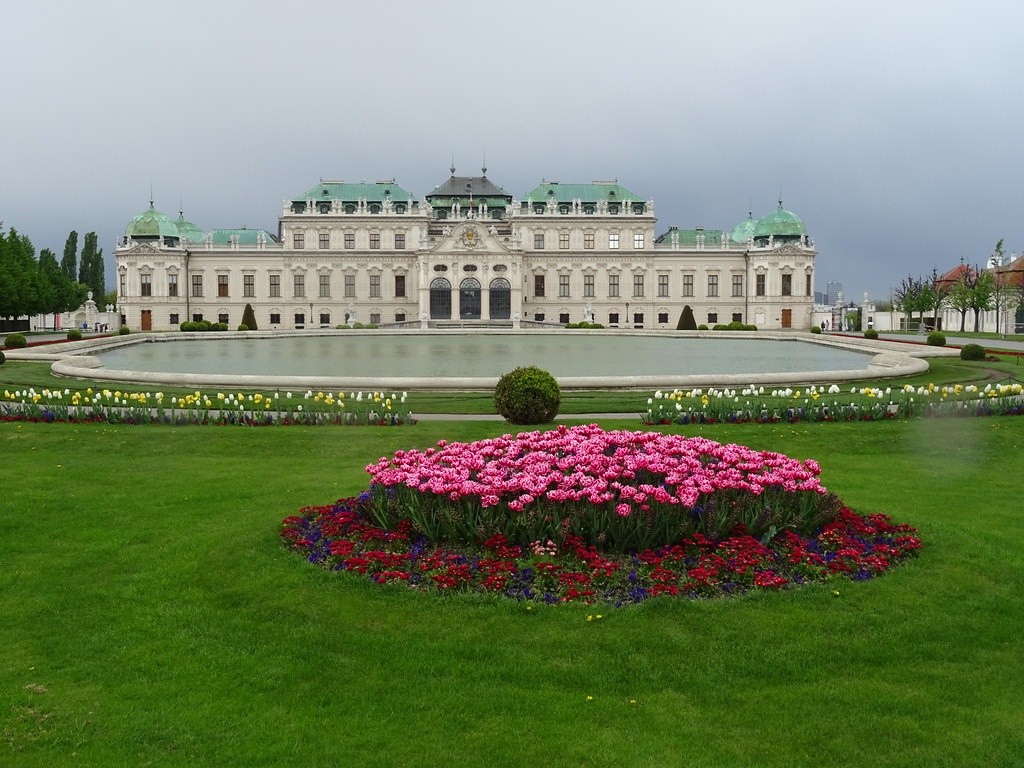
[470,200,474,208]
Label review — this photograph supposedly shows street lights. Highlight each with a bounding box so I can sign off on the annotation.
[184,248,191,323]
[742,248,749,324]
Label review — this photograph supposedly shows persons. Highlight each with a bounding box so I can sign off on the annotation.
[105,323,107,330]
[83,321,87,329]
[825,319,829,330]
[99,324,104,333]
[95,323,98,333]
[821,320,825,331]
